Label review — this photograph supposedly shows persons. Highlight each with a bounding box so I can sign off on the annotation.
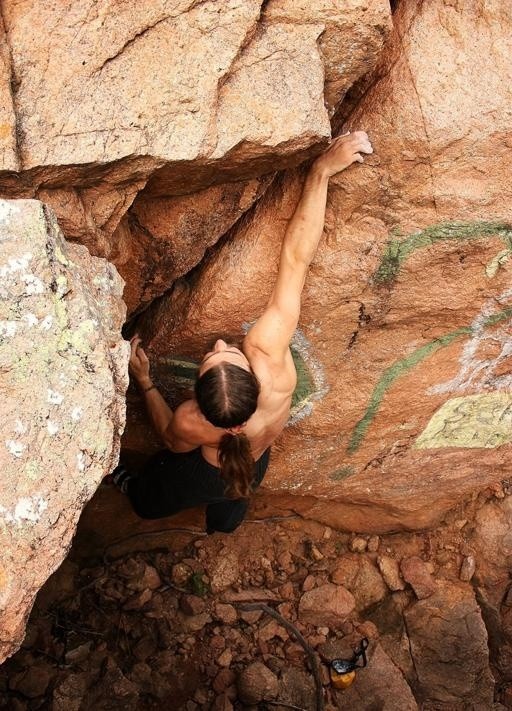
[112,130,375,536]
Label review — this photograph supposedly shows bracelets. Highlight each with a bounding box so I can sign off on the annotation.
[142,384,156,394]
